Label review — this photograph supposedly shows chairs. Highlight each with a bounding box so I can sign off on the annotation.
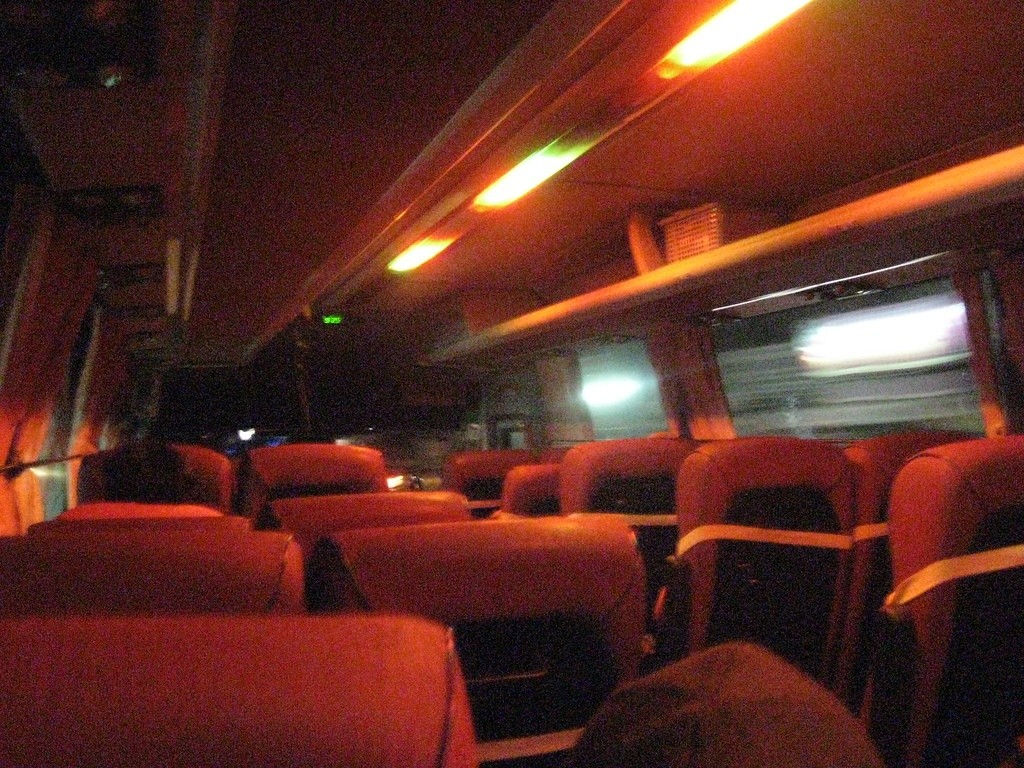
[0,426,1024,768]
